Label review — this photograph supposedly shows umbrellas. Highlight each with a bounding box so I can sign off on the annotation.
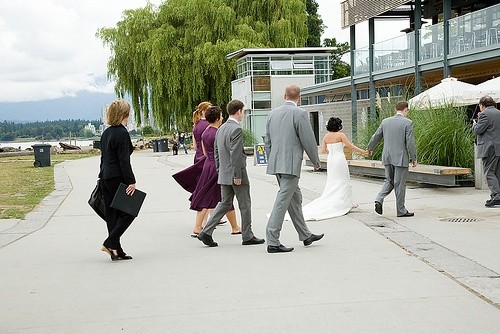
[405,78,488,110]
[464,76,500,104]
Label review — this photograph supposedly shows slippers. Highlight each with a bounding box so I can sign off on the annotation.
[231,230,242,235]
[191,232,199,238]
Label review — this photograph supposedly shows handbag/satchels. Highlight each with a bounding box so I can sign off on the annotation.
[88,180,106,222]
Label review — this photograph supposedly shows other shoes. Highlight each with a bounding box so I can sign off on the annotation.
[217,220,228,224]
[117,248,132,259]
[484,198,500,207]
[102,240,120,260]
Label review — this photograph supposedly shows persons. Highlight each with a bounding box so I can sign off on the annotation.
[265,117,369,221]
[192,102,228,227]
[197,100,265,247]
[90,100,137,261]
[366,100,417,217]
[472,95,500,207]
[172,134,180,155]
[188,106,242,237]
[265,84,324,253]
[176,131,188,155]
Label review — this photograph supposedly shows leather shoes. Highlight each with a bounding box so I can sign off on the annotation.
[197,232,218,247]
[374,202,383,215]
[303,233,324,246]
[397,211,415,217]
[267,244,295,253]
[241,236,266,245]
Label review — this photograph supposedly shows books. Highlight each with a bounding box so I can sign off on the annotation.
[110,183,147,217]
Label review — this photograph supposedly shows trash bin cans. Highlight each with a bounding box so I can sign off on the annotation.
[152,139,158,152]
[157,138,169,152]
[31,143,52,167]
